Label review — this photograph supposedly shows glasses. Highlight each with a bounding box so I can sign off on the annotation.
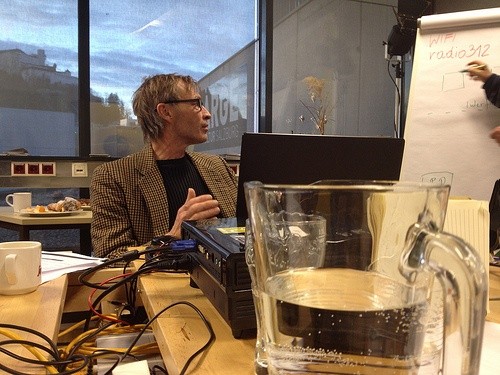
[154,97,204,111]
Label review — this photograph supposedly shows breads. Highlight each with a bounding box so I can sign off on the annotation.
[36,196,81,212]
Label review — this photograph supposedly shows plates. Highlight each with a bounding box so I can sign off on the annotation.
[20,208,83,215]
[80,206,91,210]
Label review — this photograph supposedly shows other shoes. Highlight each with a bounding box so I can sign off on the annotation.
[488,249,500,266]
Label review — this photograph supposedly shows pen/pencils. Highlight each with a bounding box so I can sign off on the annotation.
[458,64,486,73]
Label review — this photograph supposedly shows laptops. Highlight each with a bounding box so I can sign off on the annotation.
[206,133,405,250]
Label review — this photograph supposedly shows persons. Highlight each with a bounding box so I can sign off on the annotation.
[458,60,500,144]
[90,73,239,262]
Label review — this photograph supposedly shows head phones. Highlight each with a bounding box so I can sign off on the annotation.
[144,235,181,264]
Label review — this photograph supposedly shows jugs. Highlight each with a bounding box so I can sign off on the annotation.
[243,178,487,375]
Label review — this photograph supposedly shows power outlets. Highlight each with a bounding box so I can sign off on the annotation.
[72,163,88,177]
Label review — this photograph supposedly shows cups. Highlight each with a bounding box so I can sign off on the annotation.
[0,241,41,296]
[245,211,326,375]
[6,192,32,213]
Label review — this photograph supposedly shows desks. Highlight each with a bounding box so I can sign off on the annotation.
[1,246,500,375]
[0,206,94,257]
